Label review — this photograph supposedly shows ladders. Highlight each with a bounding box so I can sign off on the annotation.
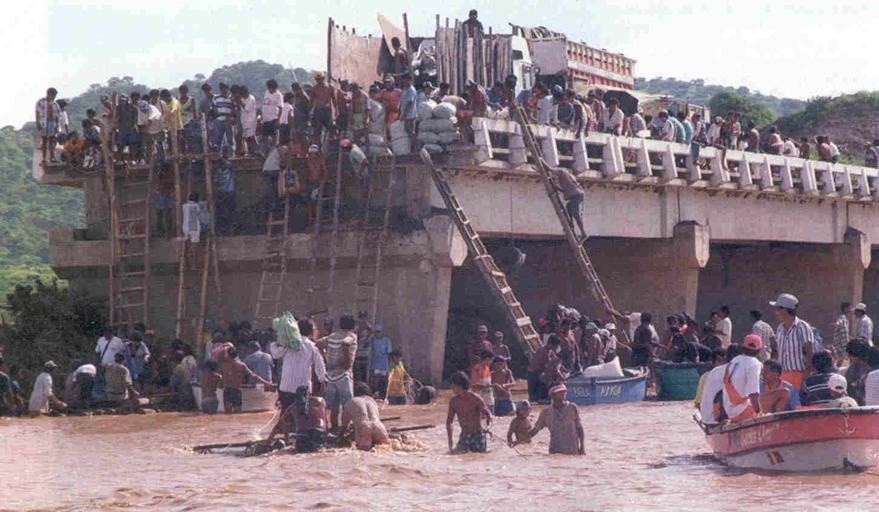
[355,152,394,384]
[117,155,153,339]
[252,156,292,329]
[304,146,341,339]
[514,102,631,342]
[432,166,543,363]
[176,157,213,353]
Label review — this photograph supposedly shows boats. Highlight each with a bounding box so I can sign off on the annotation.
[193,415,435,458]
[652,356,709,402]
[191,379,279,414]
[553,363,650,404]
[690,401,879,476]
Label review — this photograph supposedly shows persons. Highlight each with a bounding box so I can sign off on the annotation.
[30,69,467,240]
[466,72,879,199]
[461,10,485,37]
[180,190,211,274]
[389,36,412,77]
[0,289,877,455]
[539,155,587,239]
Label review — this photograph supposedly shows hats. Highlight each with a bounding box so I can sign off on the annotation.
[742,335,762,350]
[549,384,567,395]
[828,373,847,393]
[422,81,435,89]
[605,324,617,329]
[769,294,799,309]
[854,303,866,310]
